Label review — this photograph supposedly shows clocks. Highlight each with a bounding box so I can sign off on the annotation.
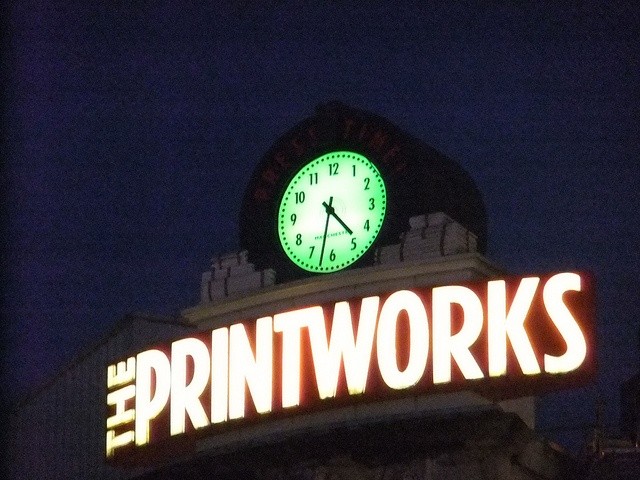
[277,150,387,275]
